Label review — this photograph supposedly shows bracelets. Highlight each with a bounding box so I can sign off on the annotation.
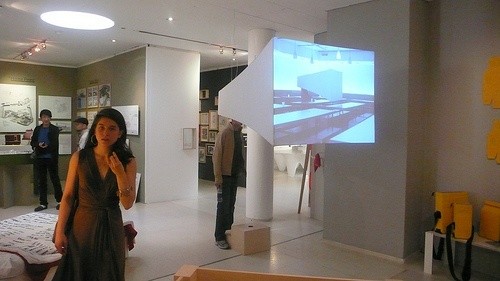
[117,185,132,197]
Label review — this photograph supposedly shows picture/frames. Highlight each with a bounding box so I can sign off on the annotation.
[199,96,247,163]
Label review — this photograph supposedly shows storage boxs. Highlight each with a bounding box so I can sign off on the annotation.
[231,222,270,254]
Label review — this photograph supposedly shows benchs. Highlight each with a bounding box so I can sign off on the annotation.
[0,209,137,281]
[423,231,500,274]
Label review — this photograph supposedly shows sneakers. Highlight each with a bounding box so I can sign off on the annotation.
[216,240,230,249]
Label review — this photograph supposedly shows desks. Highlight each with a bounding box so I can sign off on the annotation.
[274,145,305,174]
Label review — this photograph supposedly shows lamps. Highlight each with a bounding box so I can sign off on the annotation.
[19,42,47,61]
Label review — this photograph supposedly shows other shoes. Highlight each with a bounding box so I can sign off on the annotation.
[34,205,47,212]
[56,204,60,210]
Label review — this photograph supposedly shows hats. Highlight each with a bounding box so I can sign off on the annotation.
[72,118,88,126]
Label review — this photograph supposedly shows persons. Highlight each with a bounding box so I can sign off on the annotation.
[212,120,246,250]
[30,110,63,211]
[72,118,90,151]
[51,108,136,281]
